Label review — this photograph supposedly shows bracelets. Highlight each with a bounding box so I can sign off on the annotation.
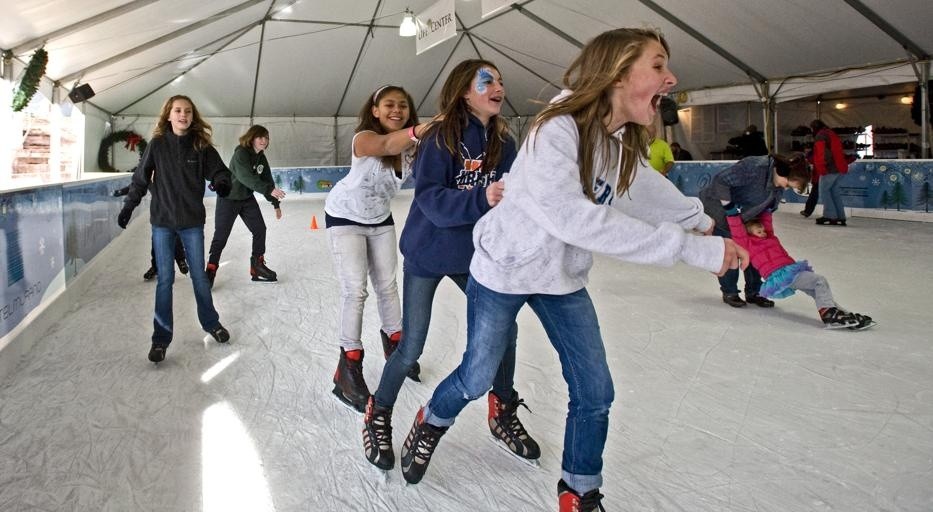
[408,125,419,142]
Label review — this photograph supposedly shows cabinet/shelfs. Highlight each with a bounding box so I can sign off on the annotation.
[791,125,921,159]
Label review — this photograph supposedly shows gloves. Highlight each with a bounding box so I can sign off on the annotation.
[215,183,230,198]
[118,209,132,229]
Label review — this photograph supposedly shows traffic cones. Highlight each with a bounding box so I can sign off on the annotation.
[310,215,318,230]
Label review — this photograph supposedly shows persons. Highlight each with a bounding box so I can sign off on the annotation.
[119,94,232,362]
[401,28,751,512]
[206,124,286,289]
[362,58,541,471]
[324,84,446,404]
[114,186,188,280]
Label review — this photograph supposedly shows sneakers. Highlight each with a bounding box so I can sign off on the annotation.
[148,342,170,361]
[747,295,775,307]
[332,348,371,402]
[816,216,831,221]
[144,267,158,279]
[207,323,230,343]
[801,211,808,216]
[177,260,189,274]
[381,330,421,378]
[722,295,746,308]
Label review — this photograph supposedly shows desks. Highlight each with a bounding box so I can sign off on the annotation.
[710,151,721,159]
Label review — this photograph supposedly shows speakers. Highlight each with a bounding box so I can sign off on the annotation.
[661,100,679,126]
[68,83,96,103]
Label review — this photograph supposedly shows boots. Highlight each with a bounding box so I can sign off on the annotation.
[362,396,395,470]
[486,391,541,460]
[557,480,607,512]
[250,254,276,279]
[205,263,219,287]
[819,308,871,329]
[401,405,450,484]
